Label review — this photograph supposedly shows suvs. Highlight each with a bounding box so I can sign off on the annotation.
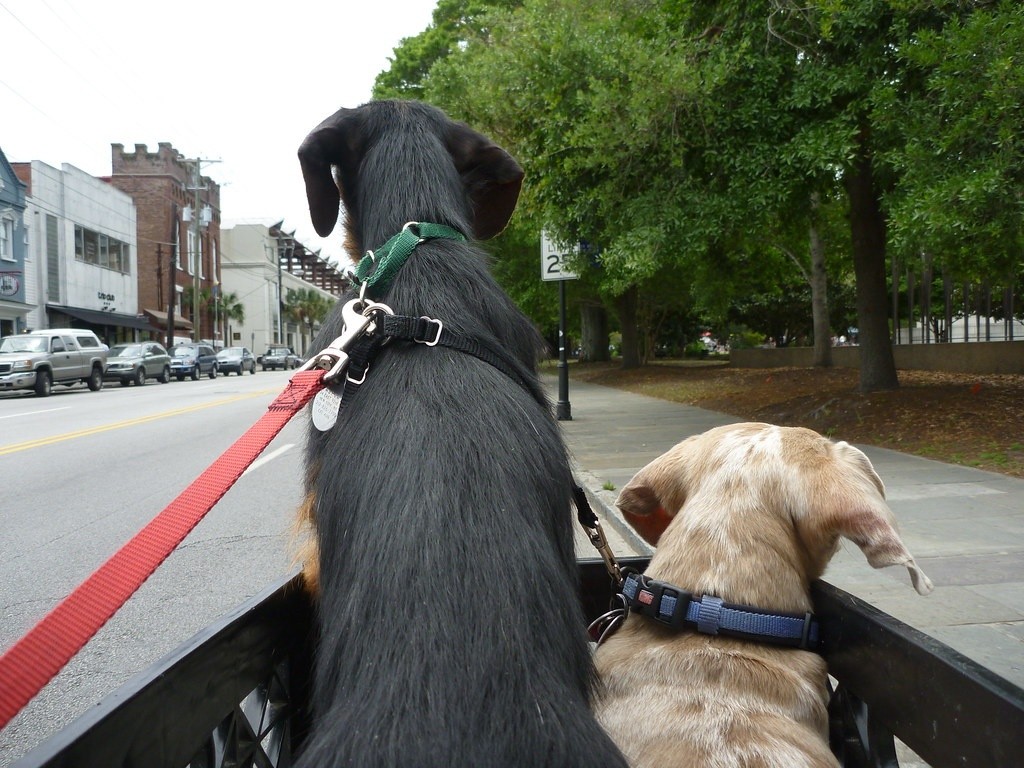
[164,343,220,381]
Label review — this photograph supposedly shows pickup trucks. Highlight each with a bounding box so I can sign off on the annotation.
[0,327,109,398]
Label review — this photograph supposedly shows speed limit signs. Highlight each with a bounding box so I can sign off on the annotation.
[539,224,582,281]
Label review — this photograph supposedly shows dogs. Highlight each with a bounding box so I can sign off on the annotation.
[297,98,936,763]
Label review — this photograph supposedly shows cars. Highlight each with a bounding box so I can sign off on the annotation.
[295,356,308,366]
[701,337,716,353]
[101,340,173,385]
[215,346,257,376]
[261,347,298,371]
